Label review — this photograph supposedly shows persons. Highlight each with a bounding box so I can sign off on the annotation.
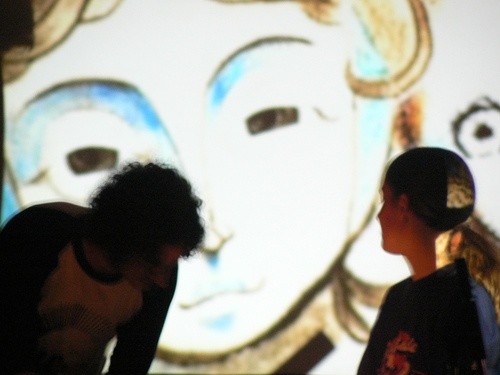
[357,147,500,375]
[0,161,206,375]
